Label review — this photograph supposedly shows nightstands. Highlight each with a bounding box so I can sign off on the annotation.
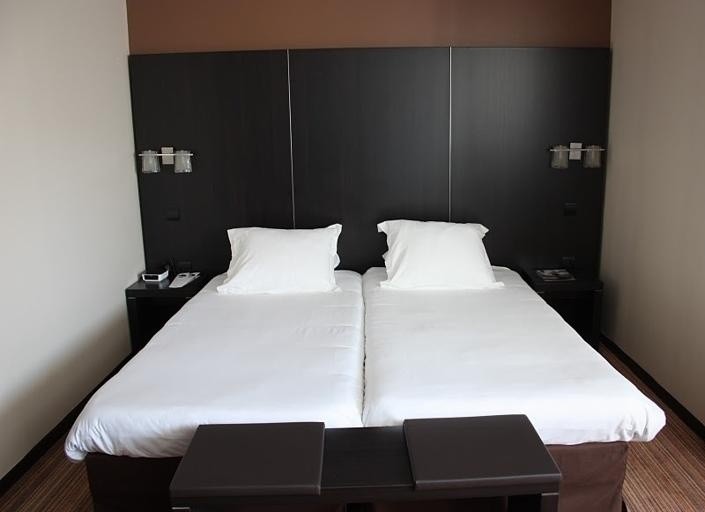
[126,274,211,356]
[523,267,602,354]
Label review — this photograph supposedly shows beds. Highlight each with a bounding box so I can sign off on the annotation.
[62,268,362,512]
[363,266,665,512]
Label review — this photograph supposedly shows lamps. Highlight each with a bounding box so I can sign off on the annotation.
[583,145,605,167]
[139,149,159,173]
[550,144,568,169]
[175,149,193,173]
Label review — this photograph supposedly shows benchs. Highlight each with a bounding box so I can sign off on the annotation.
[168,413,560,511]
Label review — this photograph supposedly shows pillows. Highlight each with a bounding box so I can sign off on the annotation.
[218,222,347,289]
[378,218,504,289]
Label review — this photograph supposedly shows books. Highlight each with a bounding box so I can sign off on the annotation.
[536,268,576,282]
